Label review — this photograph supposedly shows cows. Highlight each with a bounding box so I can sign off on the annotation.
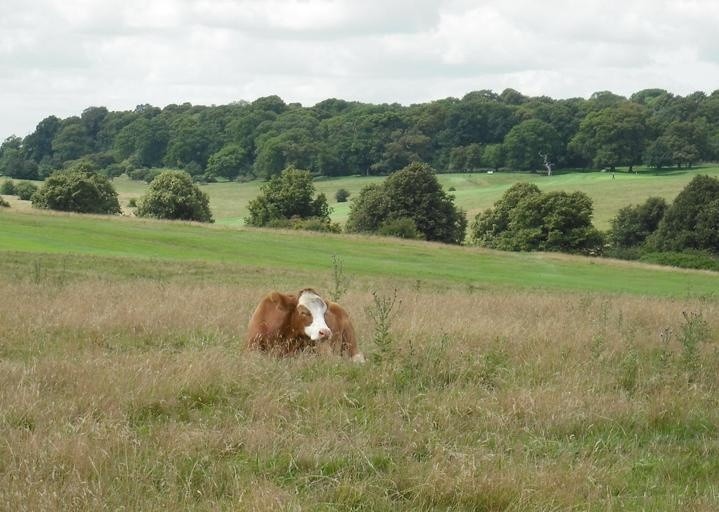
[245,287,370,367]
